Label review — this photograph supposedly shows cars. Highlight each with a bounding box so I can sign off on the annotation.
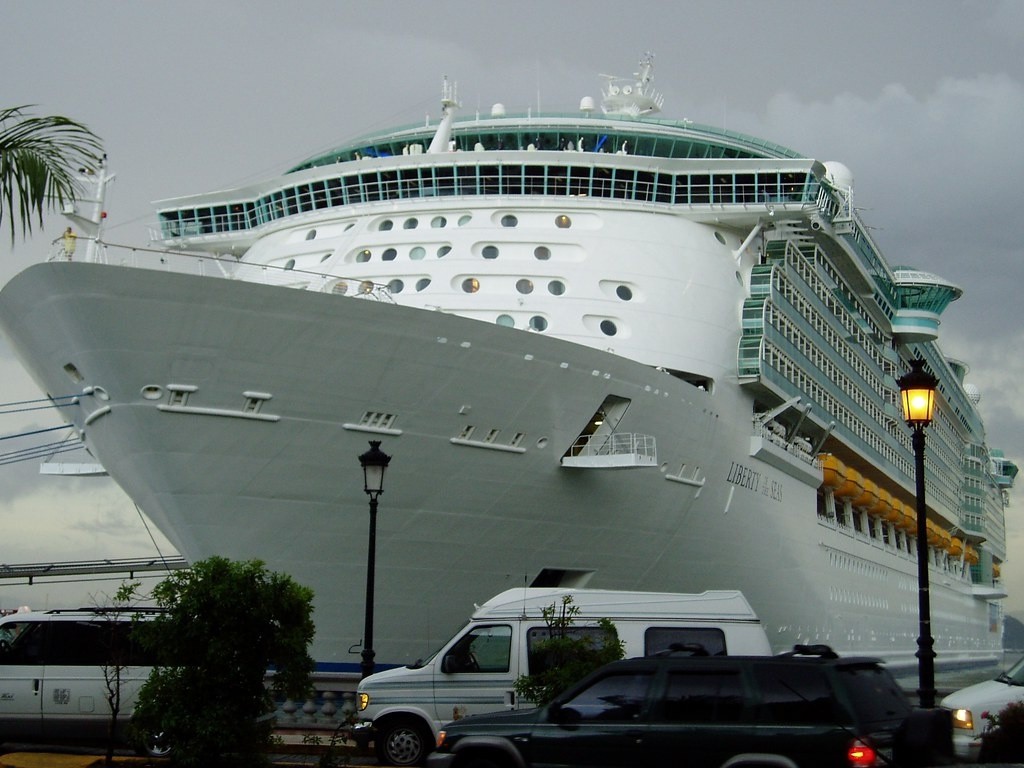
[940,656,1024,762]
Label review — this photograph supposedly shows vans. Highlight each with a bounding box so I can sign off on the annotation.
[0,605,204,759]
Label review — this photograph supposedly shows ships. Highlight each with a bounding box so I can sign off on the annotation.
[2,55,1022,692]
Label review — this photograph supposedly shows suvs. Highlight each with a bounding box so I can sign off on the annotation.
[434,642,916,768]
[352,582,775,768]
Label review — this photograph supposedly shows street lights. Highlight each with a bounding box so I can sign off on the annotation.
[894,355,942,709]
[357,438,394,752]
